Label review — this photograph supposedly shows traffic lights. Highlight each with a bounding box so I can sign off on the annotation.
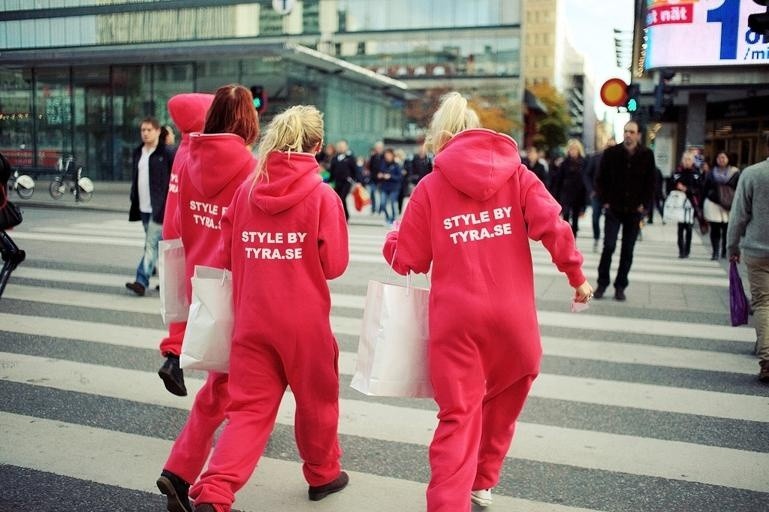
[250,85,266,113]
[625,83,640,114]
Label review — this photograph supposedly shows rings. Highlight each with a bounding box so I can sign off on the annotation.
[589,292,593,299]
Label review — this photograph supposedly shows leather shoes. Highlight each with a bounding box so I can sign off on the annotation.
[594,288,606,299]
[156,468,191,512]
[309,472,348,501]
[126,281,145,296]
[616,288,625,299]
[158,355,187,397]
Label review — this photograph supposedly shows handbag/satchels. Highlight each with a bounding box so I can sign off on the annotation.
[180,265,234,373]
[158,238,190,323]
[349,183,371,211]
[663,190,695,225]
[351,281,434,398]
[726,260,748,326]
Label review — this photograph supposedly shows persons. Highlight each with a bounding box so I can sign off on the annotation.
[726,160,768,387]
[315,139,433,223]
[0,153,22,231]
[159,94,214,395]
[382,90,594,511]
[162,124,179,166]
[159,82,259,511]
[524,121,741,301]
[124,119,169,296]
[187,105,350,512]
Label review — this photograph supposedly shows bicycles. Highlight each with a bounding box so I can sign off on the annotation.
[4,163,37,201]
[48,151,97,204]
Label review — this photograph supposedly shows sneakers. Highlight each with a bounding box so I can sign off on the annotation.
[470,487,493,508]
